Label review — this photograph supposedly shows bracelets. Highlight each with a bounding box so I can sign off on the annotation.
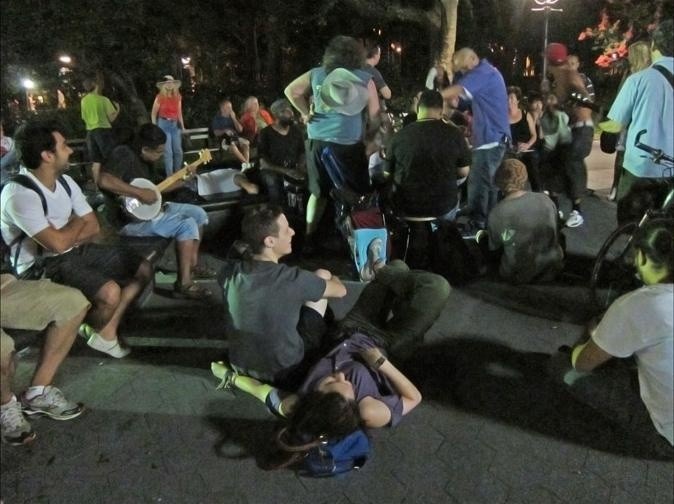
[215,370,241,393]
[370,355,387,376]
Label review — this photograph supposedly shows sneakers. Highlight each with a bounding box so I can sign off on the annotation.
[0,394,38,447]
[84,335,131,360]
[172,281,211,301]
[18,384,85,421]
[356,239,384,282]
[191,264,219,279]
[564,211,583,229]
[77,323,92,340]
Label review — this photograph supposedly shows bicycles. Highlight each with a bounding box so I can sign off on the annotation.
[588,128,674,307]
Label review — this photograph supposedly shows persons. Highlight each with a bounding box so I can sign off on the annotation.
[151,76,188,178]
[210,238,450,430]
[380,88,472,231]
[485,157,562,278]
[0,125,21,187]
[241,97,275,140]
[208,100,256,174]
[596,19,674,226]
[537,42,599,230]
[283,35,379,258]
[0,117,153,358]
[177,168,261,200]
[507,86,542,194]
[437,44,512,221]
[567,52,594,109]
[215,202,347,392]
[256,98,308,208]
[79,76,120,184]
[572,217,674,462]
[97,121,212,302]
[529,96,540,119]
[0,260,93,448]
[357,38,391,103]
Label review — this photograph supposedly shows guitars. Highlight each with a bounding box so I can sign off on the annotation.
[563,85,610,119]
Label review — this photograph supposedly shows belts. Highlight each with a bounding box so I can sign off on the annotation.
[569,119,595,128]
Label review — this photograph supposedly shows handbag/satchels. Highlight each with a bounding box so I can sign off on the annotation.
[261,424,371,478]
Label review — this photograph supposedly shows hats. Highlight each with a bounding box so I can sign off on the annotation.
[312,68,370,116]
[270,100,291,116]
[540,42,566,59]
[157,74,182,91]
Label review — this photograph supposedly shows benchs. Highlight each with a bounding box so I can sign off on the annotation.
[190,168,270,213]
[66,138,93,178]
[179,126,219,156]
[2,233,173,352]
